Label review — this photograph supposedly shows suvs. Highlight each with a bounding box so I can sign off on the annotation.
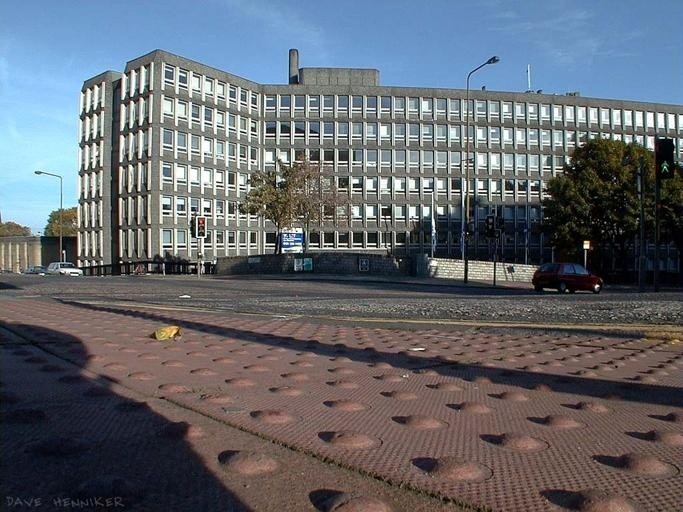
[47,262,83,277]
[530,260,604,296]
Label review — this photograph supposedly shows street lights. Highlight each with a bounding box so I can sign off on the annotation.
[459,56,501,289]
[34,170,62,262]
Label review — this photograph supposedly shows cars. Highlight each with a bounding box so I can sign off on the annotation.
[23,265,47,277]
[0,269,21,275]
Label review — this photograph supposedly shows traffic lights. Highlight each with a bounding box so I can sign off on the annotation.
[653,138,676,180]
[484,213,495,237]
[194,216,207,240]
